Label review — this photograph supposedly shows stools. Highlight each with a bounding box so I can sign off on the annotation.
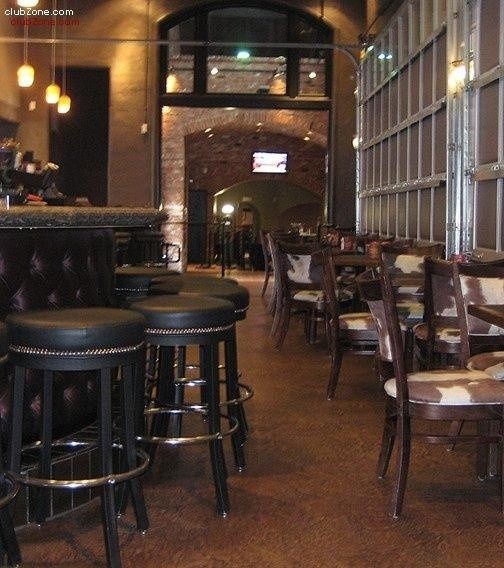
[1,264,255,568]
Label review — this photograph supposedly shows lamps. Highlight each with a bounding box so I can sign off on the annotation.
[452,58,466,97]
[17,0,71,115]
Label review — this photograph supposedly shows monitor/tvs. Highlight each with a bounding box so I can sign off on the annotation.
[252,152,287,175]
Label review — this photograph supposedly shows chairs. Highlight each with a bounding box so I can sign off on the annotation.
[259,222,504,520]
